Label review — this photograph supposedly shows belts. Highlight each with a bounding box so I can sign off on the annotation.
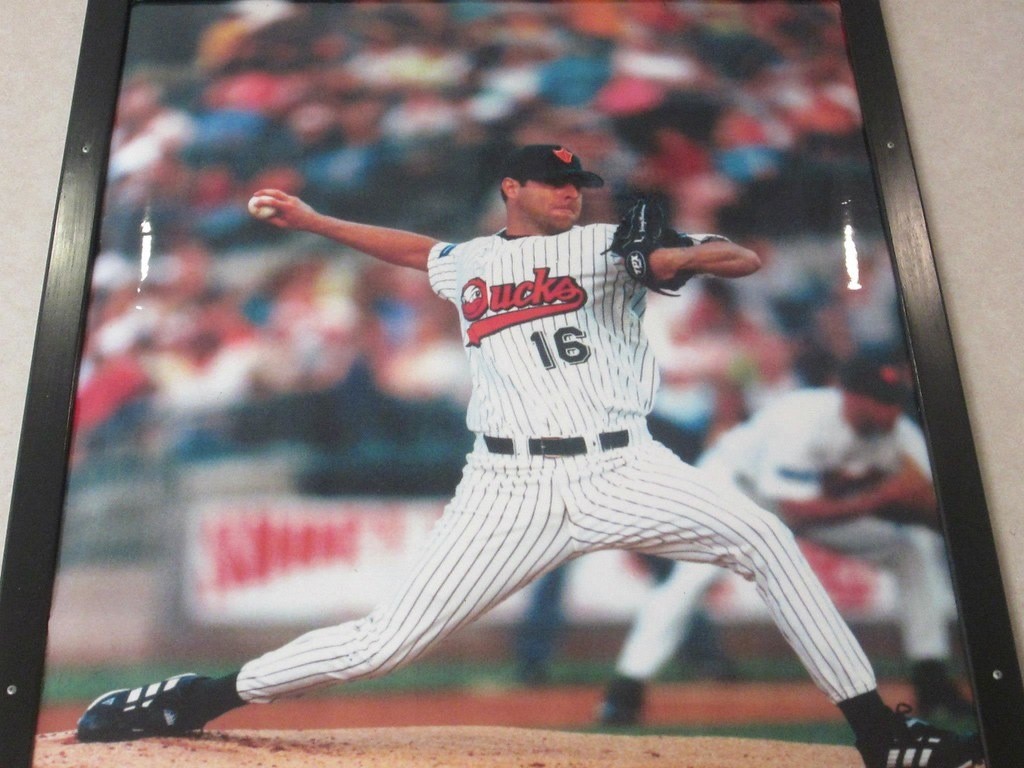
[484,429,628,459]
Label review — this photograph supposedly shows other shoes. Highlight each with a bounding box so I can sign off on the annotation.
[601,678,641,728]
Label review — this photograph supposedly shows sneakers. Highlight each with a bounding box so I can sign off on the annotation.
[855,704,974,767]
[77,673,207,740]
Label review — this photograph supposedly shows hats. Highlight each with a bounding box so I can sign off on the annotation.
[846,361,909,404]
[502,143,604,189]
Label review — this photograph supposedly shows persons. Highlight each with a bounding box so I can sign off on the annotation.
[595,352,980,720]
[61,1,975,680]
[78,143,986,768]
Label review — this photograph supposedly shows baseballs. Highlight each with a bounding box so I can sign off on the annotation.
[248,196,276,220]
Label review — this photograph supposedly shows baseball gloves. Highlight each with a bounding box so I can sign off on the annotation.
[612,197,695,292]
